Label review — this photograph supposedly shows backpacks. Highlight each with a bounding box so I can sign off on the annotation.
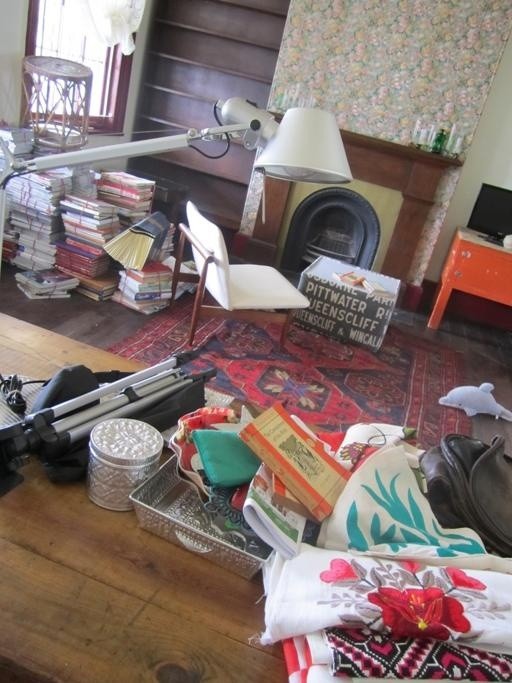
[417,431,512,560]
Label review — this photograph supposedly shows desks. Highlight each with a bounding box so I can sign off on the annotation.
[426,225,512,330]
[0,311,285,682]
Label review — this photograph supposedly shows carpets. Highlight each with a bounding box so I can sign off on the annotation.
[106,288,473,446]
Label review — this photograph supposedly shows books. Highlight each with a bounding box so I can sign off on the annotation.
[334,268,391,297]
[237,398,354,523]
[1,125,203,316]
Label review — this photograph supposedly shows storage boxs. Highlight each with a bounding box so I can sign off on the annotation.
[291,253,403,355]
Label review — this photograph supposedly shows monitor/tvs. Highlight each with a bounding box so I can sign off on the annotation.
[466,182,512,247]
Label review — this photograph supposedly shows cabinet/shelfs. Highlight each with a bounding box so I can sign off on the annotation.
[127,1,293,233]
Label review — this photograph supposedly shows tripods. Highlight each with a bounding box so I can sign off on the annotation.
[24,349,217,453]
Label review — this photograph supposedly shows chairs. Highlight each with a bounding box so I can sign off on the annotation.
[168,199,311,354]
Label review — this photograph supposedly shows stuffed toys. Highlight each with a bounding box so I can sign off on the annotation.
[438,381,512,424]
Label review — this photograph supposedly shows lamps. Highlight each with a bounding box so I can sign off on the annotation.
[0,96,356,258]
